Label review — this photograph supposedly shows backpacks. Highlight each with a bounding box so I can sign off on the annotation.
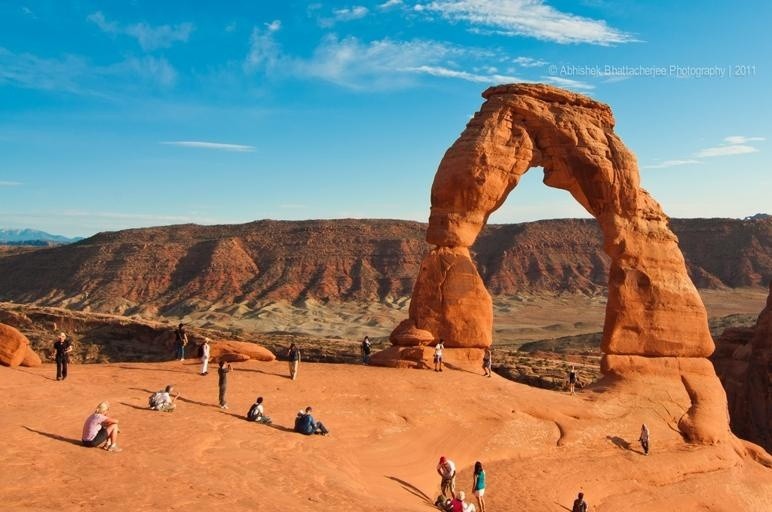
[149,391,169,406]
[247,404,260,421]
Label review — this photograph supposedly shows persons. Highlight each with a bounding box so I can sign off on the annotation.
[435,456,486,512]
[482,347,492,377]
[362,336,371,366]
[569,366,577,395]
[638,424,649,455]
[82,402,122,452]
[571,493,587,512]
[148,323,328,435]
[51,332,72,381]
[433,339,446,372]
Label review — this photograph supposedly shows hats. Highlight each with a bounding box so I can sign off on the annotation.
[456,490,465,500]
[439,457,447,465]
[306,406,312,412]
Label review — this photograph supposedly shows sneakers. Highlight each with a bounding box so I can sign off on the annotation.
[57,375,66,381]
[103,443,123,453]
[219,404,228,409]
